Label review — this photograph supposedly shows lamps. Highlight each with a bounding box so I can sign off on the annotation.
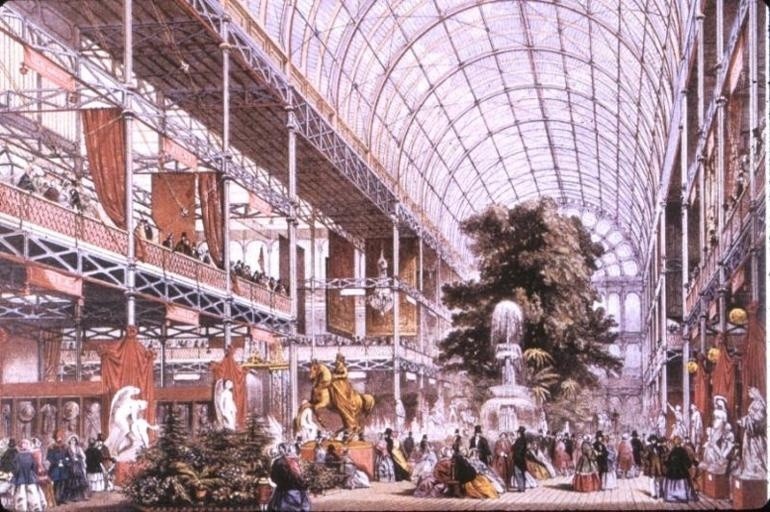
[364,249,396,317]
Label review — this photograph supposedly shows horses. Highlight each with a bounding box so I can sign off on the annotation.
[295,359,376,442]
